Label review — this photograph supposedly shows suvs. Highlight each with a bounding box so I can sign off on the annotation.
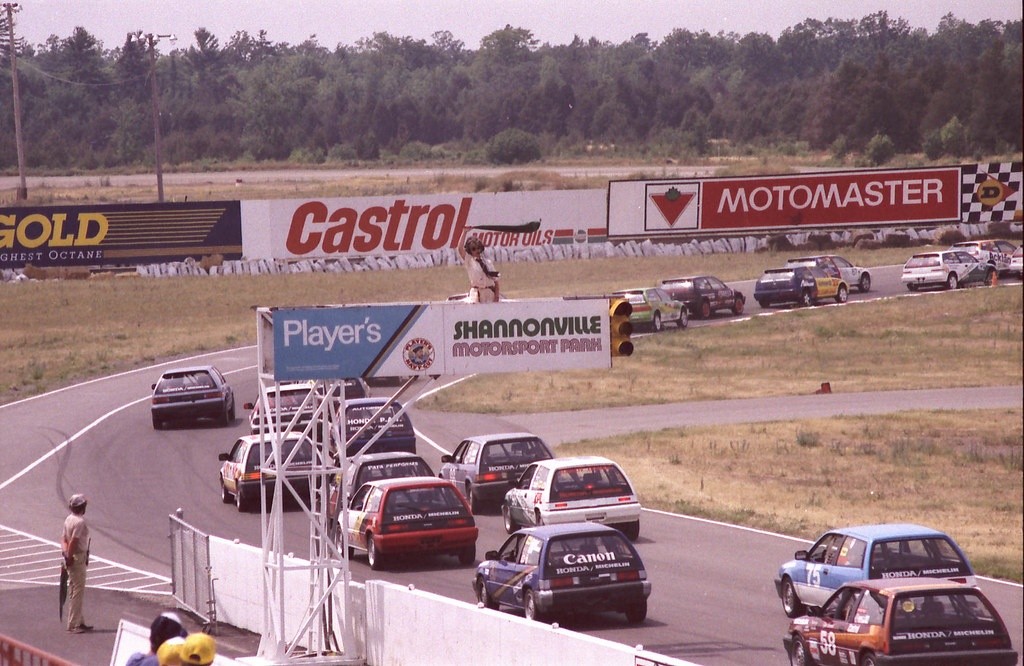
[659,274,745,319]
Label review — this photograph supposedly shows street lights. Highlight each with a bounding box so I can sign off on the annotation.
[146,32,177,201]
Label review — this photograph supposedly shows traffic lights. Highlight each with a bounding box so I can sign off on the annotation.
[610,298,634,358]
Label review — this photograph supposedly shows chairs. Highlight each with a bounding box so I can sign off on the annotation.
[510,443,529,463]
[171,375,185,389]
[580,543,600,557]
[919,601,950,627]
[582,472,604,487]
[197,375,209,386]
[417,491,440,510]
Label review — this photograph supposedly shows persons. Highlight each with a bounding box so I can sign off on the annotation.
[458,225,501,303]
[60,493,94,634]
[126,610,184,666]
[157,632,215,666]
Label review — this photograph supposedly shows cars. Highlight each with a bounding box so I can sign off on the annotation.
[318,450,438,526]
[780,575,1019,666]
[786,254,871,292]
[900,250,1000,292]
[1008,244,1023,279]
[333,474,479,571]
[150,364,236,430]
[217,431,331,514]
[471,521,652,626]
[772,521,982,620]
[611,287,687,332]
[216,367,415,447]
[948,239,1018,279]
[438,430,559,514]
[444,291,507,302]
[499,455,641,547]
[753,265,850,309]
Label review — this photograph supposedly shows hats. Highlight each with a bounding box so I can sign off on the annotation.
[151,611,187,644]
[179,633,216,665]
[156,636,185,666]
[69,494,87,507]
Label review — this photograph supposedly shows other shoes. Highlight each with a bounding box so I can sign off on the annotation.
[80,623,93,630]
[68,627,85,634]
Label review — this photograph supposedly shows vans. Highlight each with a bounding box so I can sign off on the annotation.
[323,397,417,467]
